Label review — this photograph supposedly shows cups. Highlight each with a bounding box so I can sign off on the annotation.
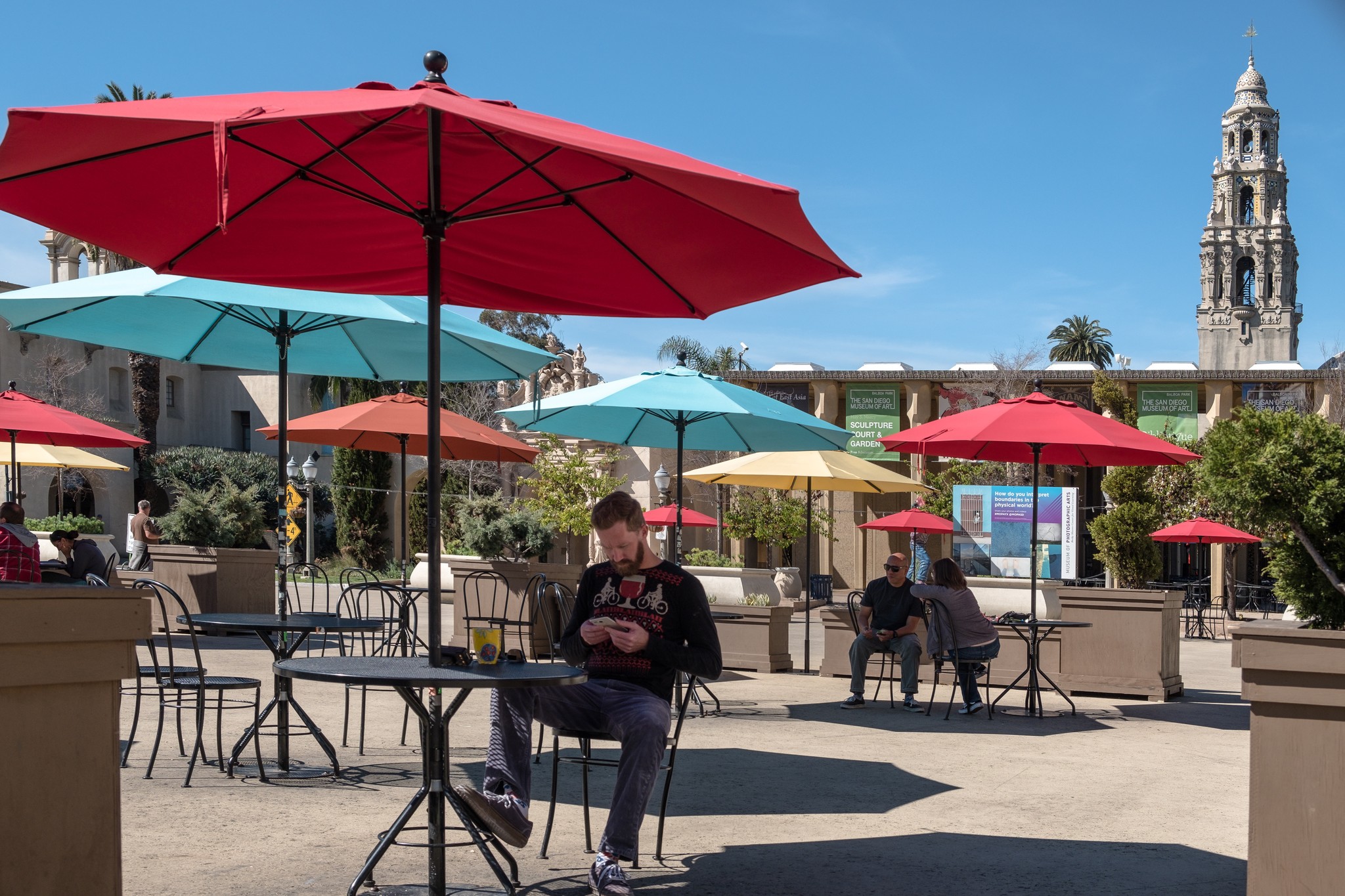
[472,627,502,664]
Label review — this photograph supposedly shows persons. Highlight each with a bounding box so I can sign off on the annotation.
[906,501,932,585]
[840,553,925,713]
[0,501,41,583]
[910,557,1000,714]
[128,500,166,572]
[454,491,723,896]
[96,514,103,521]
[41,530,108,586]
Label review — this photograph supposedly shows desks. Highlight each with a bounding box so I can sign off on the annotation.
[348,582,456,658]
[983,614,1096,718]
[1183,601,1222,640]
[38,558,67,571]
[269,656,592,896]
[175,608,385,779]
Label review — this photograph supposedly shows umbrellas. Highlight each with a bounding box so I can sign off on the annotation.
[642,499,731,564]
[495,352,856,717]
[0,40,863,896]
[0,381,149,505]
[877,378,1203,721]
[858,502,968,585]
[0,268,561,779]
[1148,513,1264,639]
[675,449,941,675]
[254,382,542,655]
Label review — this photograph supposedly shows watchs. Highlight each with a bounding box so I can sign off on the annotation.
[893,630,898,638]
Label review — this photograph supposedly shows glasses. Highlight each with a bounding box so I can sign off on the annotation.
[884,564,908,572]
[931,570,936,577]
[498,649,526,664]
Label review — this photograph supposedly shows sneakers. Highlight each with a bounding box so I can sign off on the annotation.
[959,699,984,714]
[903,699,924,712]
[451,783,532,847]
[588,861,634,896]
[840,696,865,709]
[974,664,988,678]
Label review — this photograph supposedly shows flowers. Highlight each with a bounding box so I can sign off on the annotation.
[15,490,26,495]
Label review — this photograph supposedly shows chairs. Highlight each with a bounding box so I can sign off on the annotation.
[488,571,547,663]
[1064,579,1288,642]
[921,596,1000,720]
[553,581,594,773]
[333,581,424,758]
[338,567,398,658]
[101,551,118,586]
[537,580,584,764]
[0,548,35,583]
[847,591,923,709]
[284,561,344,659]
[535,637,699,872]
[84,570,211,767]
[131,576,272,789]
[462,569,509,656]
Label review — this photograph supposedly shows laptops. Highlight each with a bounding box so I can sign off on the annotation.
[40,561,62,565]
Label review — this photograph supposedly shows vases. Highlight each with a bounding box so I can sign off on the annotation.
[16,493,27,499]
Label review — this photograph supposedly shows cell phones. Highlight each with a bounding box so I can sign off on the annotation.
[589,617,628,633]
